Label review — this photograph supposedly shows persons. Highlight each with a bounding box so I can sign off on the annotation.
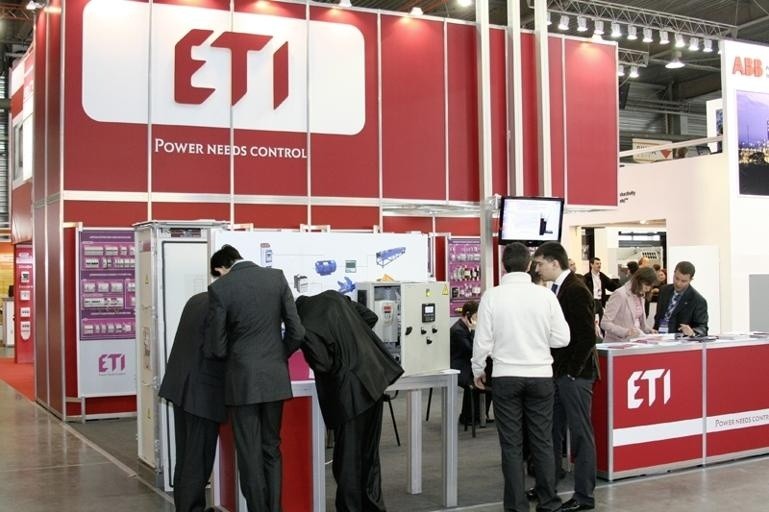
[295,290,404,512]
[568,256,709,342]
[471,242,601,512]
[158,291,229,512]
[205,244,306,512]
[449,300,495,427]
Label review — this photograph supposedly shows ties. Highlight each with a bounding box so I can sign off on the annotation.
[551,283,558,293]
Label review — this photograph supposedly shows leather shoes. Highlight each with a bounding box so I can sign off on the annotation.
[560,495,595,511]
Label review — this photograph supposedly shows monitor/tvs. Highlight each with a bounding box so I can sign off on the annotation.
[498,196,565,247]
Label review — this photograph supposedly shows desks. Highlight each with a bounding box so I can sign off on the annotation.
[209,368,460,512]
